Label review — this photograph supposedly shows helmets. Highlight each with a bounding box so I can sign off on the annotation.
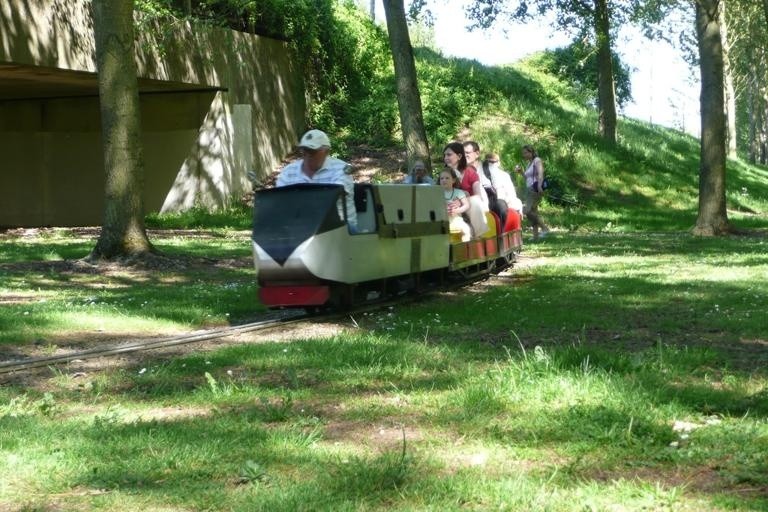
[296,129,331,150]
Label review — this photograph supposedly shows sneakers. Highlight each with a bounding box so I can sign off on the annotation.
[529,230,550,240]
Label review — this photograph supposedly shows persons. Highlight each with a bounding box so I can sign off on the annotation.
[401,140,525,242]
[515,144,550,242]
[275,129,359,234]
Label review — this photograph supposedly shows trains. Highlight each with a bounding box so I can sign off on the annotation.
[250,181,524,315]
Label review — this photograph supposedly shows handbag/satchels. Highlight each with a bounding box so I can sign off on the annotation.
[534,179,548,191]
